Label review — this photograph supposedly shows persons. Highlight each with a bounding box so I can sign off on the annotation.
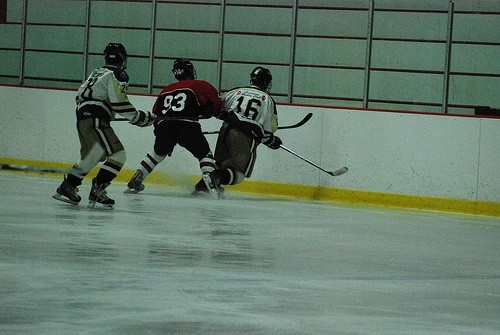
[123,57,283,194]
[51,42,154,209]
[192,65,279,199]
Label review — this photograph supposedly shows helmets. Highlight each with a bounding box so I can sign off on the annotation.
[249,65,272,92]
[103,41,128,67]
[171,57,198,81]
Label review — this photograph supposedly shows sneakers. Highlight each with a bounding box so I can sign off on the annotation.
[191,171,220,194]
[124,168,145,194]
[52,172,82,205]
[88,177,115,210]
[202,169,226,202]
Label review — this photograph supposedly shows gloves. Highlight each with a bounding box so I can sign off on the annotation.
[274,135,283,150]
[129,110,158,128]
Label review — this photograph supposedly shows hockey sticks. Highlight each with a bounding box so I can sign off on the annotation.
[201,112,318,135]
[109,116,202,123]
[278,144,347,177]
